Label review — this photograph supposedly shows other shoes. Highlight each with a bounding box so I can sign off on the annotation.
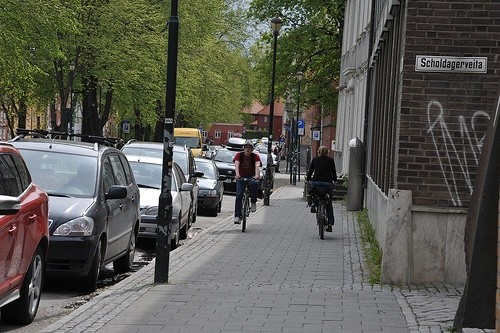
[328,226,332,232]
[234,216,241,224]
[251,203,256,212]
[311,205,316,212]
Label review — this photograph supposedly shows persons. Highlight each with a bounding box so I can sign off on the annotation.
[233,140,262,224]
[274,147,277,154]
[277,146,281,162]
[306,146,337,232]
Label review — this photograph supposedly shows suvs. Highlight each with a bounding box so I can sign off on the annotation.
[0,141,50,327]
[6,128,140,296]
[118,139,205,223]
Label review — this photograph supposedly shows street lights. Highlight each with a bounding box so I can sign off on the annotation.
[293,71,304,187]
[263,10,284,206]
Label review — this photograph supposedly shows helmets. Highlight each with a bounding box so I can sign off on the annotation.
[244,141,254,149]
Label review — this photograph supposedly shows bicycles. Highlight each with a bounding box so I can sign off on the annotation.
[306,181,333,240]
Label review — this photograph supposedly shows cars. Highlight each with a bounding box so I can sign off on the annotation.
[124,155,194,251]
[211,150,241,191]
[226,137,283,182]
[193,157,228,216]
[204,146,218,158]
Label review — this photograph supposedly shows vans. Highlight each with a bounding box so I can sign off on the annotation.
[173,129,207,158]
